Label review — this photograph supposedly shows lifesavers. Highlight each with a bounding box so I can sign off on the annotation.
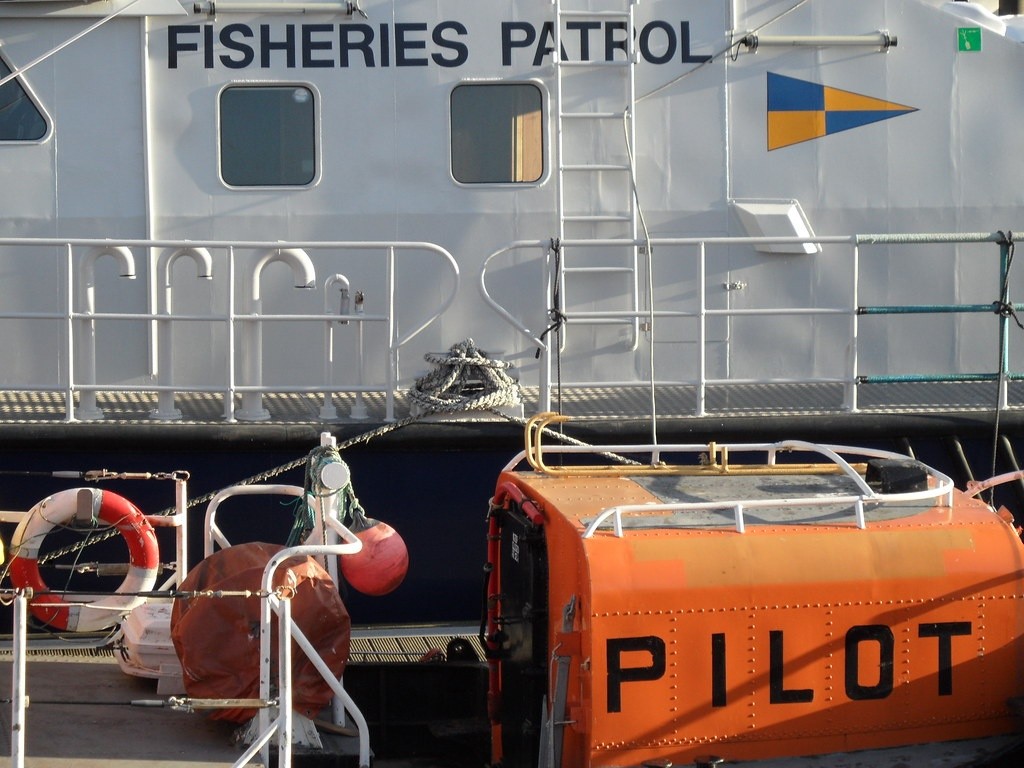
[10,484,162,640]
